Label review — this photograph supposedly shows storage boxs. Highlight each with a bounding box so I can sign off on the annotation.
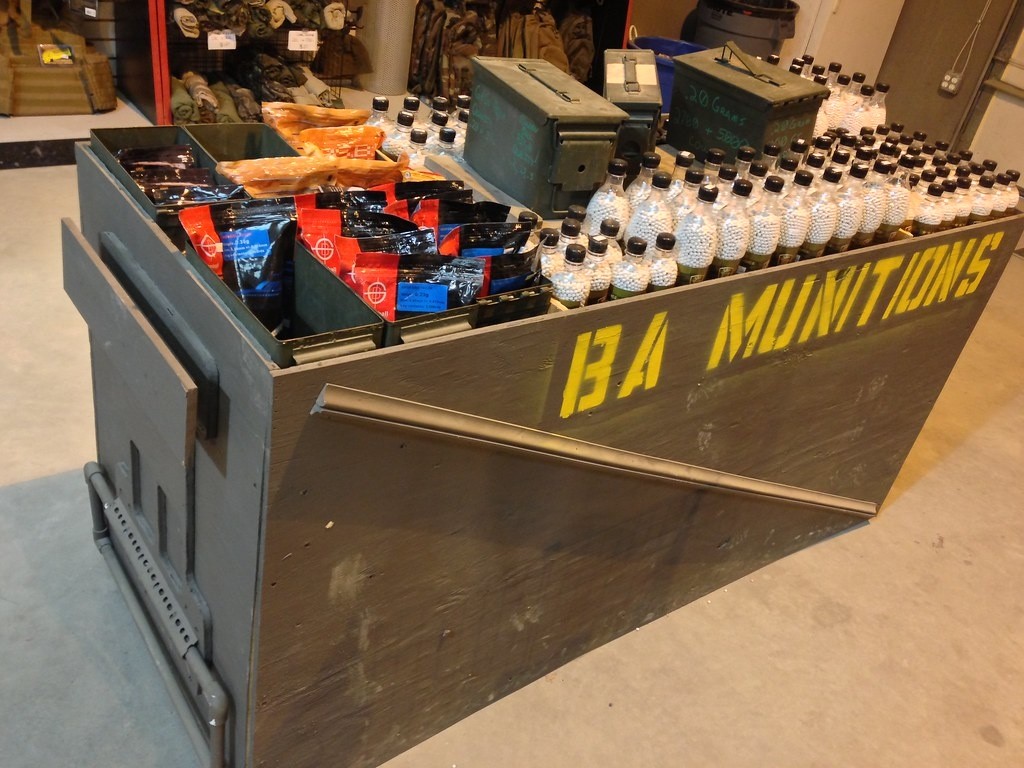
[87,122,556,369]
[602,50,664,176]
[460,55,630,219]
[666,39,831,164]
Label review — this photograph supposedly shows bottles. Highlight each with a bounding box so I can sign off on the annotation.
[366,94,471,169]
[521,53,1020,310]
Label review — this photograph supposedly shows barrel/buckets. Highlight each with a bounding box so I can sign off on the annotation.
[628,36,711,112]
